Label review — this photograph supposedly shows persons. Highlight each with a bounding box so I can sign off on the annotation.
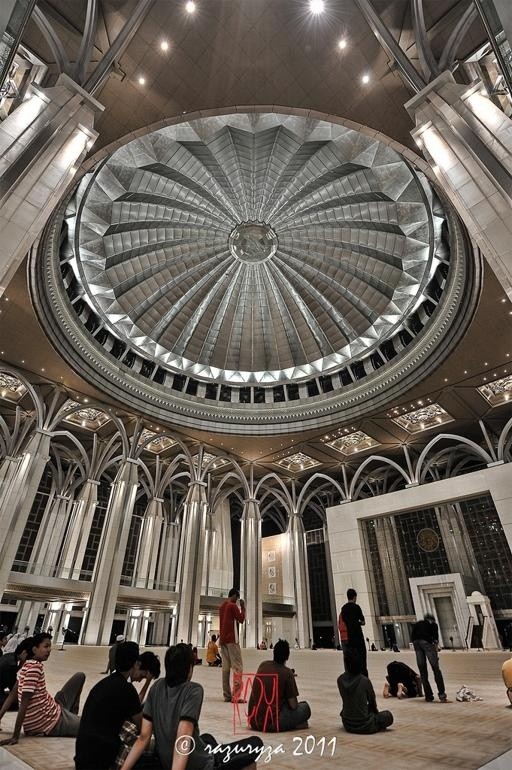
[500,656,512,709]
[1,622,459,770]
[409,614,449,704]
[336,612,347,673]
[341,589,369,681]
[218,588,250,705]
